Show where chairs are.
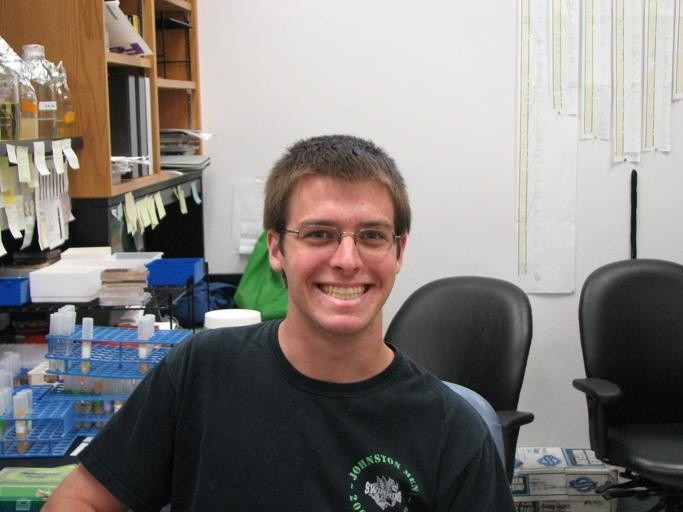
[572,260,683,512]
[384,277,534,486]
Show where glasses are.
[287,225,400,254]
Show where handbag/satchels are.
[235,230,286,320]
[169,282,236,324]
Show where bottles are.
[0,43,58,142]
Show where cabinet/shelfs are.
[0,0,204,199]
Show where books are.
[161,132,211,170]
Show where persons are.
[39,133,518,512]
